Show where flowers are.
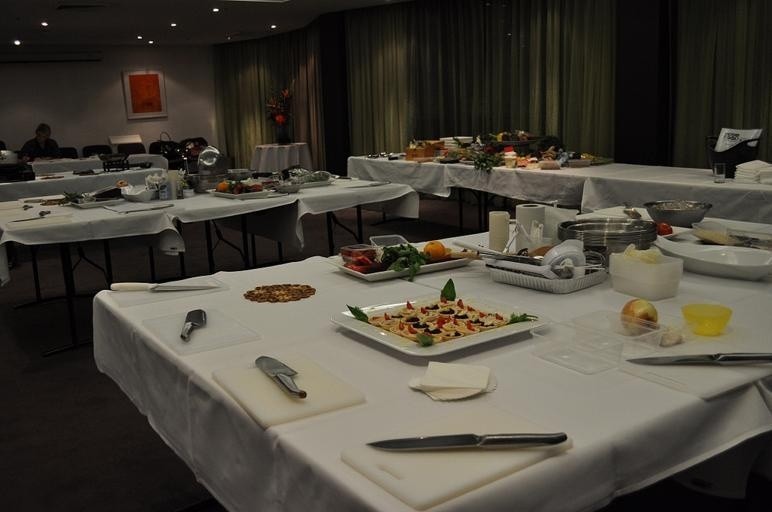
[263,88,294,123]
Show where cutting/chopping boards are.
[108,276,230,307]
[593,205,648,218]
[620,336,772,399]
[5,215,71,230]
[141,307,260,356]
[211,354,365,427]
[0,202,22,211]
[340,402,574,509]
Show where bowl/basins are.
[644,201,713,228]
[680,305,734,339]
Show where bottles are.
[158,168,168,199]
[505,146,517,168]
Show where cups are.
[714,162,727,182]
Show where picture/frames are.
[122,68,168,120]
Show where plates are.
[301,178,335,187]
[440,135,472,154]
[654,235,772,279]
[205,189,274,201]
[121,185,153,202]
[74,195,124,208]
[558,218,657,259]
[329,289,547,358]
[323,254,471,282]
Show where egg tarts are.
[367,297,514,347]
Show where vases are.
[275,123,289,145]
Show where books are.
[714,126,763,152]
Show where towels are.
[734,159,772,185]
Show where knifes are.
[110,282,220,292]
[181,308,206,342]
[255,356,307,402]
[346,180,391,190]
[623,199,641,219]
[624,351,772,367]
[366,432,569,451]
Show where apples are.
[621,298,659,336]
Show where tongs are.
[388,152,404,160]
[460,248,542,265]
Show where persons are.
[18,123,59,163]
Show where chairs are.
[181,137,208,157]
[82,145,113,158]
[117,143,146,155]
[59,146,78,159]
